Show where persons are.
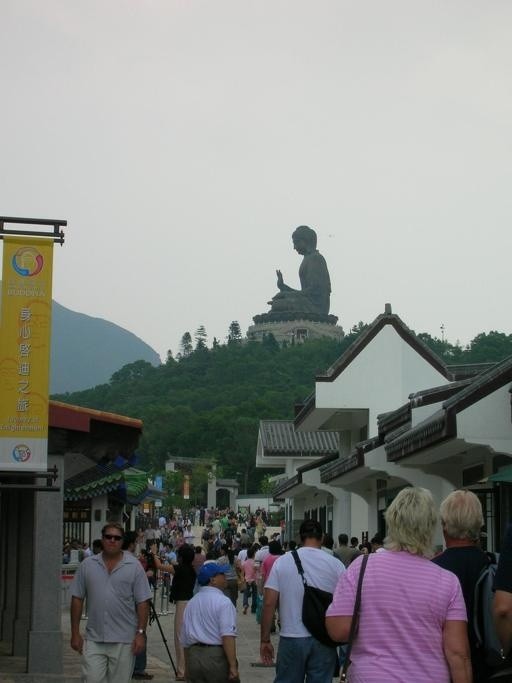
[62,504,364,683]
[269,225,332,313]
[325,487,510,683]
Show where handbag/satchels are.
[237,576,246,592]
[301,585,348,648]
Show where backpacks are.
[471,550,510,668]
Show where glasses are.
[102,534,122,540]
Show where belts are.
[193,642,224,647]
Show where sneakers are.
[242,605,248,614]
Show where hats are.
[197,560,232,584]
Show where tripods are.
[147,582,177,677]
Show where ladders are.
[362,531,368,543]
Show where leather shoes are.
[132,671,154,680]
